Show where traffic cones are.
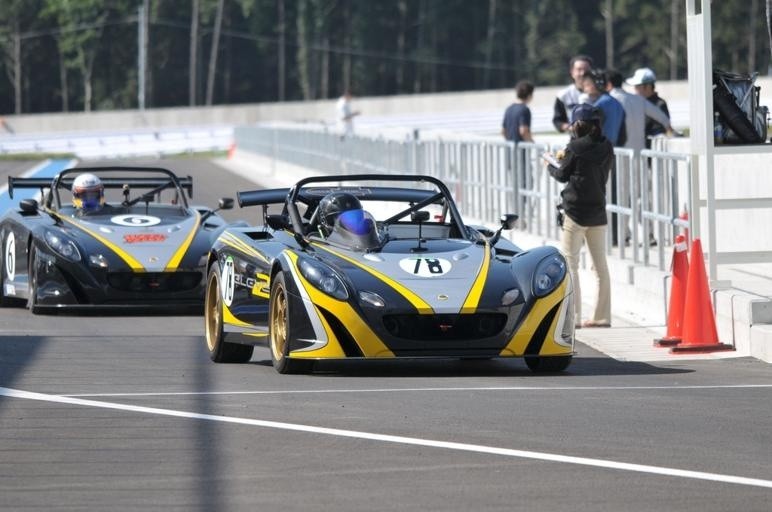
[672,237,735,352]
[654,235,700,346]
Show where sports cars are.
[1,164,248,314]
[200,174,581,376]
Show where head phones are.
[591,68,606,93]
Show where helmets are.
[315,191,362,237]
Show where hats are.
[625,68,656,86]
[567,104,600,127]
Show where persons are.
[316,190,367,244]
[603,71,684,244]
[554,58,595,134]
[538,102,614,328]
[626,68,670,166]
[574,71,626,248]
[502,81,546,208]
